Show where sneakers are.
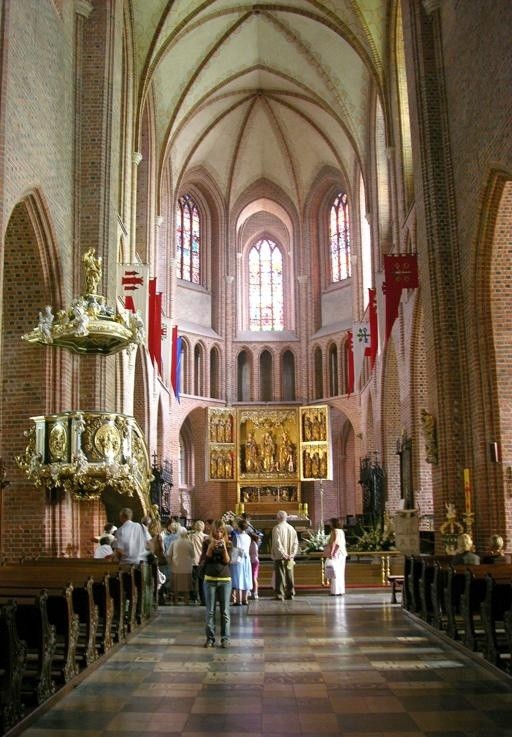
[222,642,230,648]
[204,643,214,648]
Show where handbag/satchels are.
[323,544,340,557]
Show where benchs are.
[388,552,512,666]
[0,557,150,732]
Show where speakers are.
[489,442,499,462]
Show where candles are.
[234,502,240,514]
[303,503,308,514]
[297,501,303,514]
[462,467,472,515]
[320,478,323,484]
[239,503,244,513]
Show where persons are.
[81,246,102,295]
[38,305,55,344]
[198,519,232,649]
[449,533,480,566]
[211,416,328,503]
[323,518,347,596]
[270,510,299,601]
[482,534,504,564]
[93,507,263,606]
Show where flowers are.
[349,521,396,551]
[299,528,328,553]
[220,509,236,522]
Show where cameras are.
[217,541,224,549]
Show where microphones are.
[417,502,420,511]
